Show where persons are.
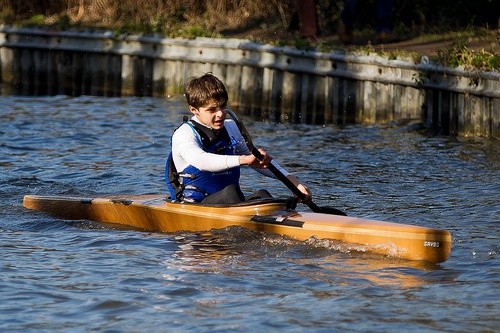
[164,73,313,210]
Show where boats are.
[23,193,450,264]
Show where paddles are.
[226,109,348,216]
